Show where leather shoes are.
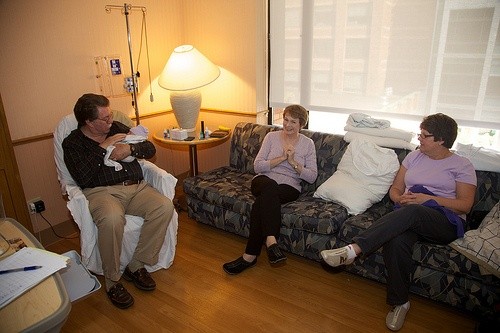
[105,282,134,309]
[122,262,156,291]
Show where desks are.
[0,217,103,333]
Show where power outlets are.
[26,197,42,215]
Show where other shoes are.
[386,300,411,331]
[222,254,257,275]
[321,246,356,268]
[266,243,289,264]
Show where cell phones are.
[184,137,195,141]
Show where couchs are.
[183,118,500,325]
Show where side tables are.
[152,129,232,211]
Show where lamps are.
[159,44,221,131]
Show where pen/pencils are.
[0,265,43,275]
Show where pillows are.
[313,140,401,216]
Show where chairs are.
[53,110,180,278]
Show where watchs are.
[129,143,135,154]
[293,164,299,169]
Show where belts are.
[113,178,143,186]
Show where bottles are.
[163,126,188,140]
[199,126,211,140]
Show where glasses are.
[96,111,113,122]
[417,133,435,139]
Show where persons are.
[222,106,319,275]
[320,113,477,331]
[61,94,175,308]
